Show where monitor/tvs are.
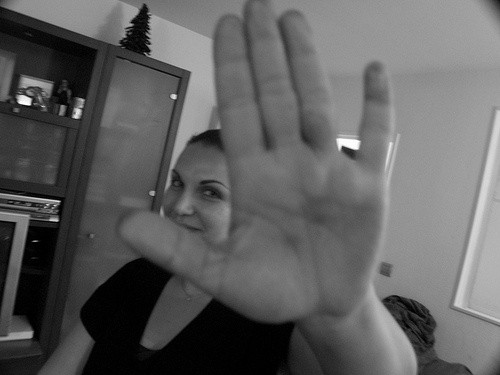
[0,211,29,337]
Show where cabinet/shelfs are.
[0,7,191,375]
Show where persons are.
[385,293,475,375]
[38,0,419,375]
